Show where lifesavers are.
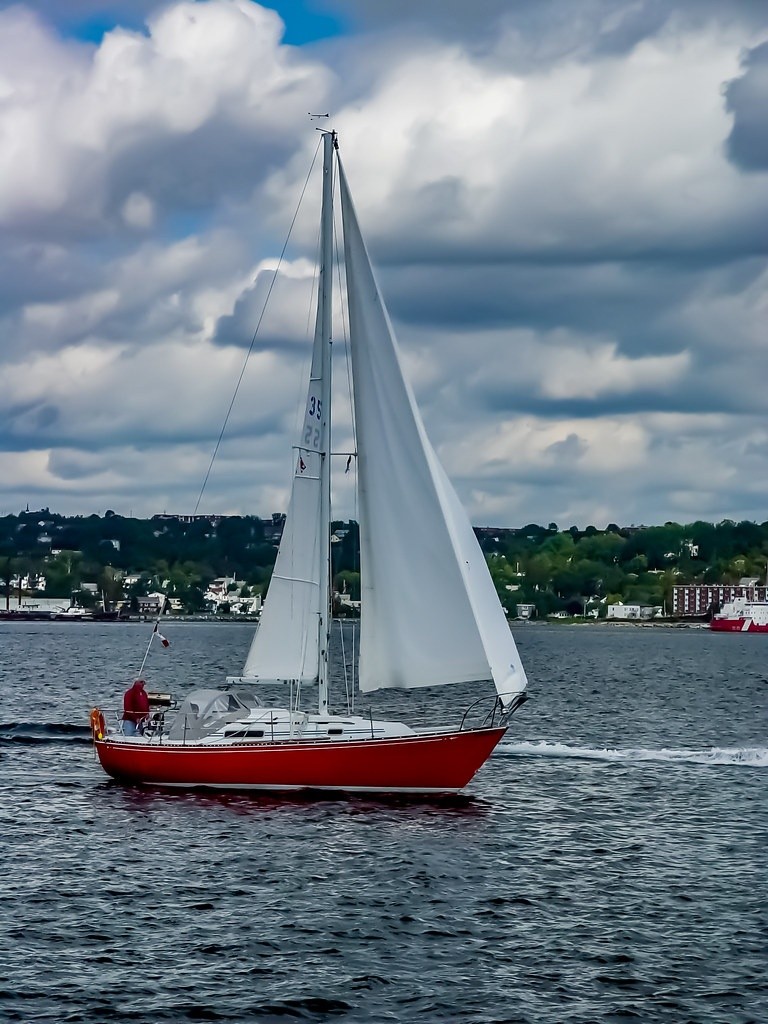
[90,708,105,739]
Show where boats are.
[0,602,122,622]
[710,594,768,632]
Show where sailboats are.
[89,112,531,795]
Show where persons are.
[119,678,151,738]
